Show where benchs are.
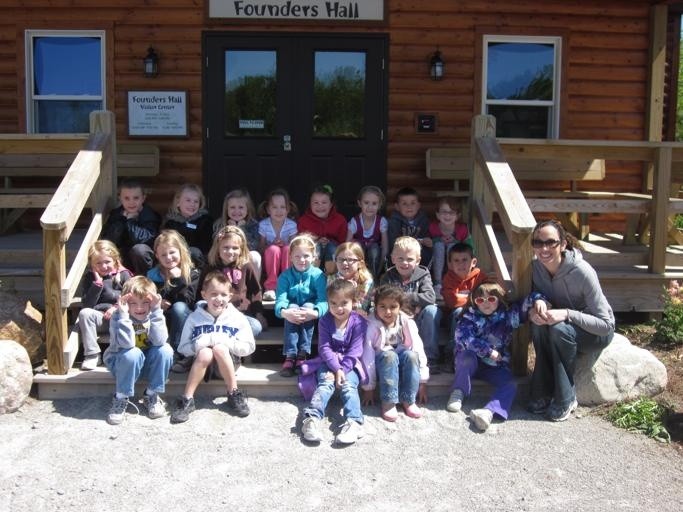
[0,133,159,240]
[427,146,606,237]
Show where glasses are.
[475,296,498,304]
[530,238,558,249]
[336,256,359,263]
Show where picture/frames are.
[126,88,190,138]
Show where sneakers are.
[335,417,363,444]
[227,388,250,417]
[446,388,465,411]
[428,356,455,374]
[170,394,195,423]
[526,394,552,414]
[470,407,493,431]
[434,283,444,300]
[171,355,193,373]
[280,350,309,378]
[143,388,166,418]
[107,391,129,424]
[381,401,398,422]
[400,398,422,418]
[263,289,276,301]
[81,353,103,369]
[545,395,579,422]
[300,416,323,441]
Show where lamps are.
[429,44,444,80]
[143,47,158,77]
